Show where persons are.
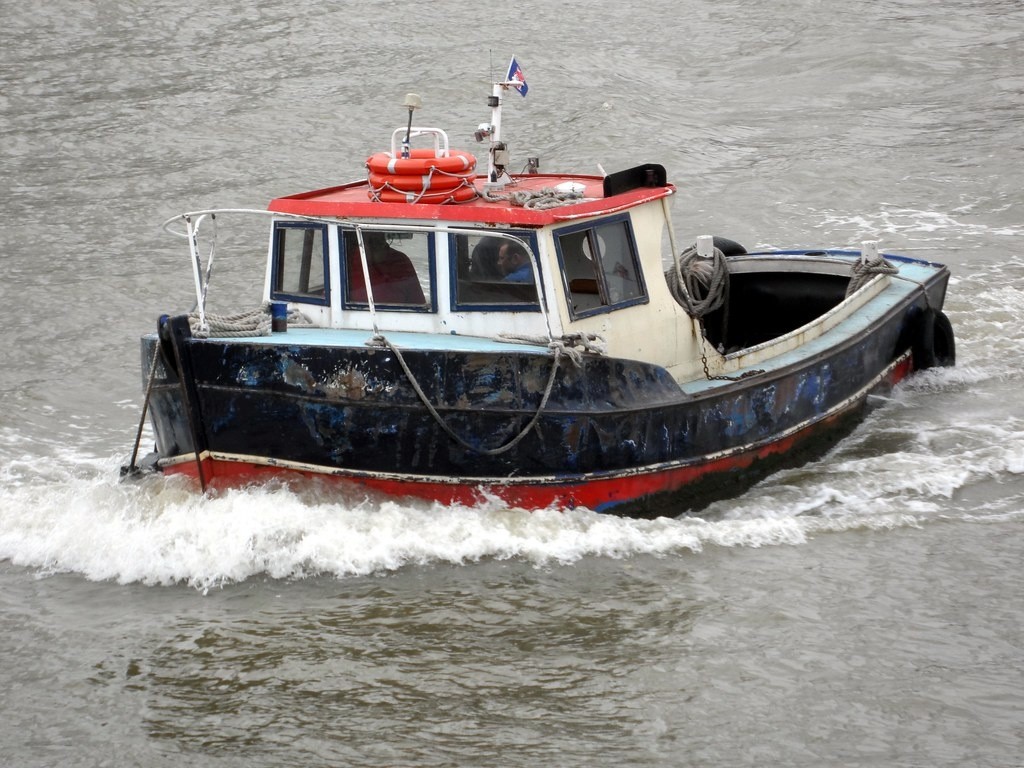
[347,231,428,313]
[498,242,535,283]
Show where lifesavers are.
[365,170,476,190]
[680,235,747,256]
[368,187,477,205]
[365,148,477,175]
[912,308,955,370]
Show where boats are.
[118,49,956,525]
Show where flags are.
[509,59,529,97]
